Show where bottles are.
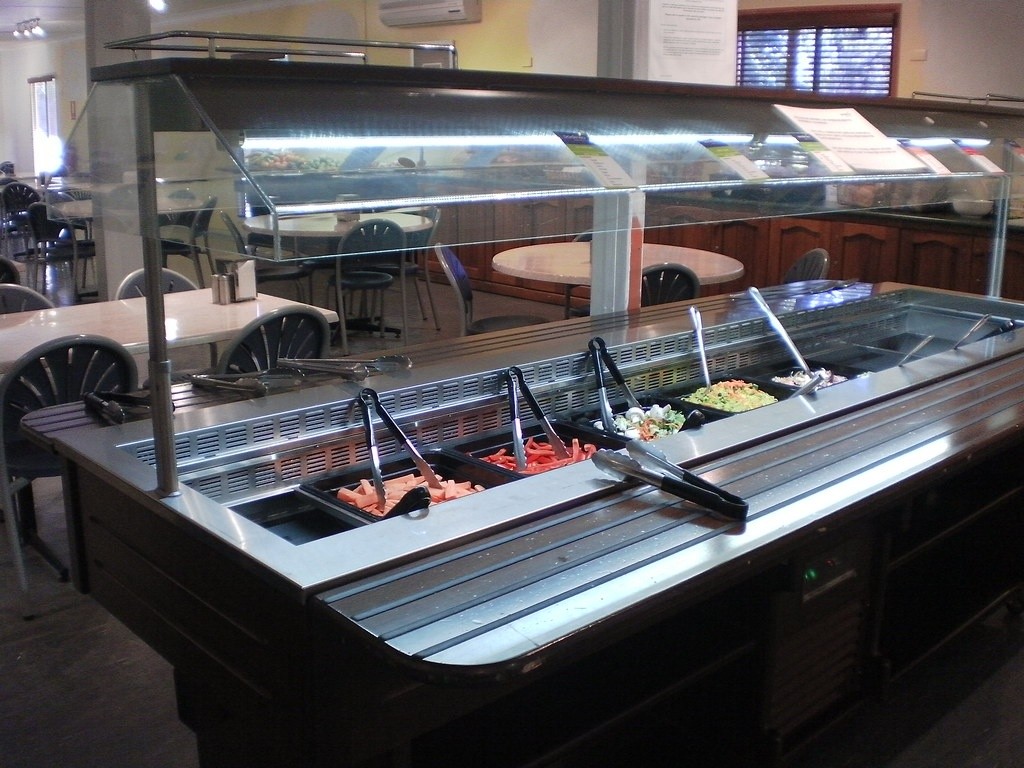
[211,274,234,305]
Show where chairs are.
[140,305,331,389]
[0,332,138,481]
[219,210,315,305]
[782,246,831,285]
[359,205,441,332]
[562,229,594,319]
[433,243,550,337]
[326,218,408,355]
[641,262,702,307]
[0,171,218,315]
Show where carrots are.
[483,435,596,473]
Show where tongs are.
[277,355,412,382]
[976,320,1016,341]
[84,391,176,425]
[382,486,432,520]
[358,388,443,514]
[588,337,642,433]
[678,410,705,433]
[505,367,570,469]
[592,439,749,519]
[190,368,307,398]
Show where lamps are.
[12,17,40,37]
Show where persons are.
[0,161,19,191]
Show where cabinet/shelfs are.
[415,181,1024,312]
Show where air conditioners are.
[378,0,482,28]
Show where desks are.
[0,176,85,260]
[491,240,744,318]
[51,197,203,297]
[240,214,433,339]
[0,288,340,584]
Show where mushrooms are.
[625,407,645,422]
[650,404,671,420]
